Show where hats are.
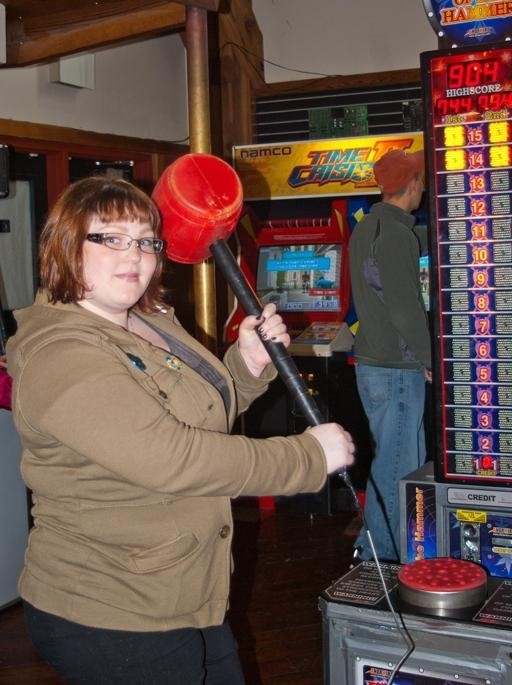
[372,148,425,193]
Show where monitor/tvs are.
[419,255,430,312]
[256,243,343,313]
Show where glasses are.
[81,230,165,256]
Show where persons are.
[301,271,311,294]
[3,173,359,685]
[314,276,334,300]
[418,267,429,292]
[347,146,433,574]
